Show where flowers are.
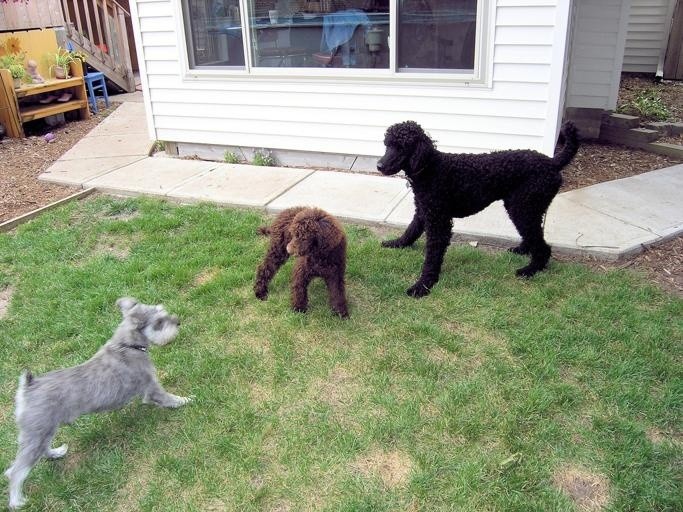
[0,35,33,84]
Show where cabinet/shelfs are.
[0,26,91,138]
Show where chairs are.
[256,26,312,66]
[309,22,364,68]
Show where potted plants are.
[45,46,87,79]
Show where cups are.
[268,10,279,25]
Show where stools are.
[83,72,110,114]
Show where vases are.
[12,77,20,88]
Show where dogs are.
[252,204,350,321]
[374,119,583,300]
[3,295,198,512]
